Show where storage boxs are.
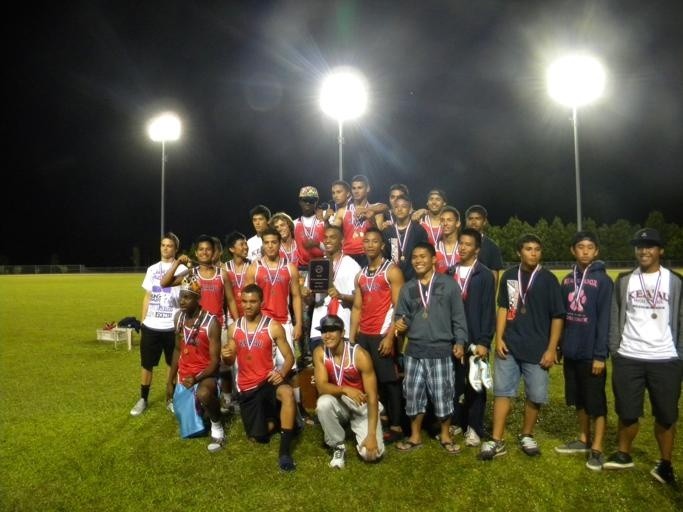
[97,327,128,341]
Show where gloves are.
[555,346,563,364]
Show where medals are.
[651,313,657,319]
[520,307,527,314]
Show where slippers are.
[208,430,225,451]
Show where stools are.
[113,325,141,352]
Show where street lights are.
[314,60,372,184]
[140,106,183,242]
[545,50,611,227]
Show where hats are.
[631,228,662,246]
[573,231,598,246]
[300,186,318,198]
[180,277,202,296]
[314,314,344,330]
[428,188,447,200]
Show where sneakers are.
[279,455,296,472]
[384,425,480,454]
[296,401,315,425]
[220,393,240,414]
[555,439,634,470]
[130,398,147,416]
[518,431,541,456]
[167,403,175,414]
[469,355,493,392]
[651,458,678,491]
[478,439,507,459]
[328,442,345,469]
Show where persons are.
[552,232,615,472]
[476,234,567,460]
[129,175,503,471]
[600,226,682,494]
